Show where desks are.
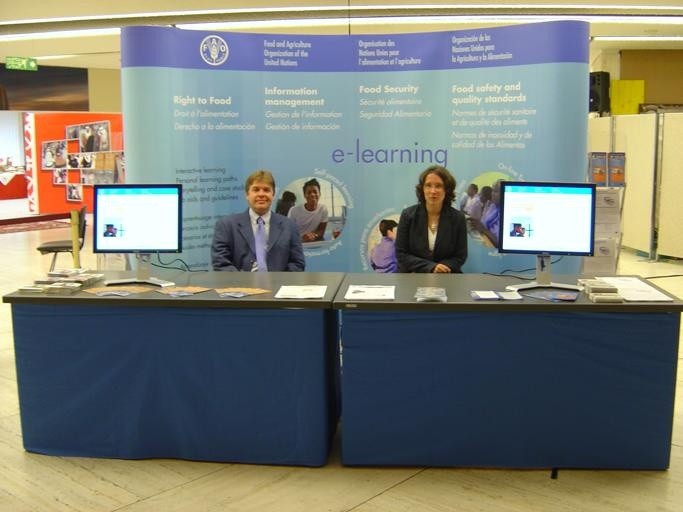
[3,270,346,467]
[333,272,683,470]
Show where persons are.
[287,179,329,244]
[209,169,306,273]
[508,222,528,237]
[275,191,296,216]
[462,178,503,248]
[103,224,117,237]
[393,163,469,273]
[41,121,125,205]
[367,218,399,273]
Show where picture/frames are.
[41,120,124,201]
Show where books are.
[579,284,622,304]
[576,276,619,294]
[16,268,176,302]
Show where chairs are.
[36,206,87,272]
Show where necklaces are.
[425,206,440,232]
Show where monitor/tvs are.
[93,184,182,288]
[498,181,596,291]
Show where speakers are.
[589,71,610,112]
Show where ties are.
[255,218,267,272]
